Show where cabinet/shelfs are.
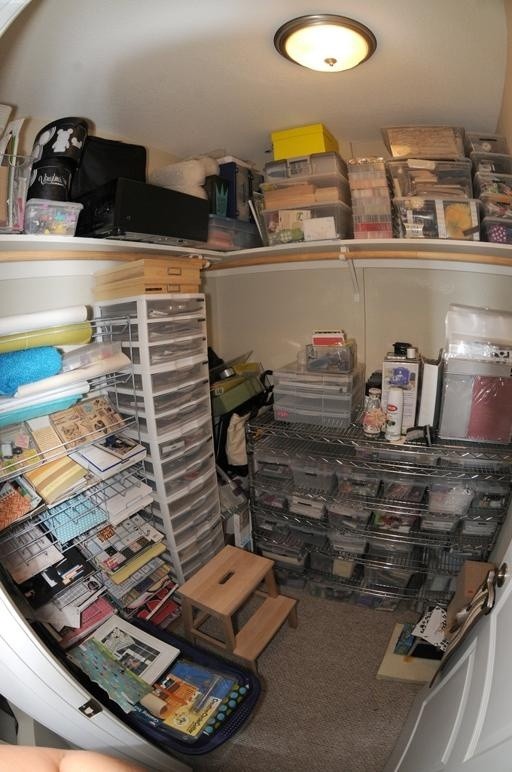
[247,301,512,609]
[0,295,260,772]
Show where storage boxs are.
[0,103,512,302]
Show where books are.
[27,437,149,502]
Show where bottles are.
[384,386,404,441]
[363,387,383,438]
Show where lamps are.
[275,15,377,73]
